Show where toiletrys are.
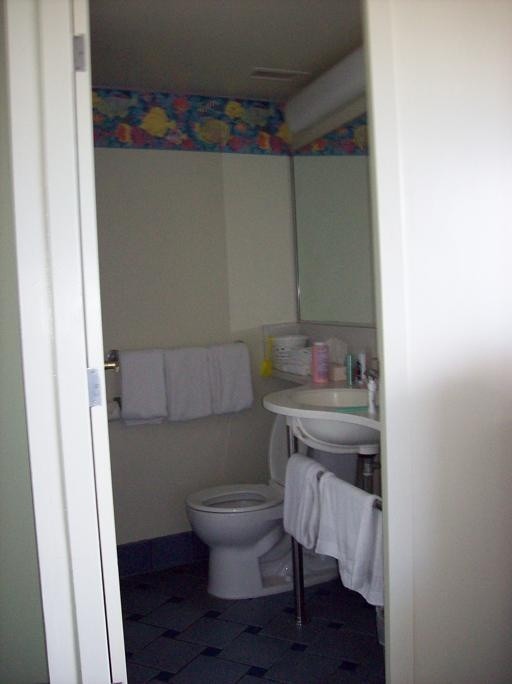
[367,375,377,415]
[346,351,368,384]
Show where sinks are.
[263,387,379,456]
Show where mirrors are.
[291,111,374,329]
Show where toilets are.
[185,414,358,600]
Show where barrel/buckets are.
[270,334,310,369]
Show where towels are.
[118,337,253,428]
[281,452,385,606]
